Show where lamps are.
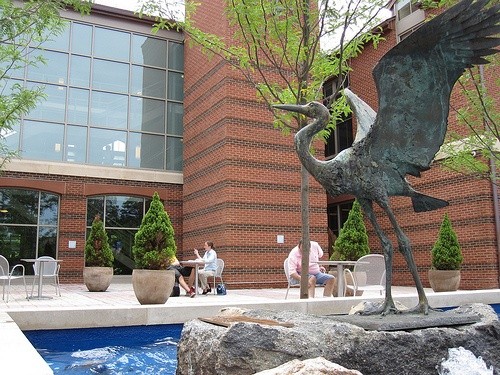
[0,193,10,212]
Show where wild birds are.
[270,0,500,318]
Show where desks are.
[18,258,63,300]
[180,260,214,297]
[310,260,370,297]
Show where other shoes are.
[202,285,212,295]
[186,287,196,296]
[187,288,196,298]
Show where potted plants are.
[328,204,368,296]
[83,213,113,291]
[429,213,463,293]
[132,191,176,304]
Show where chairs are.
[284,258,328,299]
[31,256,61,297]
[0,255,29,303]
[344,254,388,297]
[212,259,226,295]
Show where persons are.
[288,239,335,297]
[170,256,196,298]
[193,241,217,294]
